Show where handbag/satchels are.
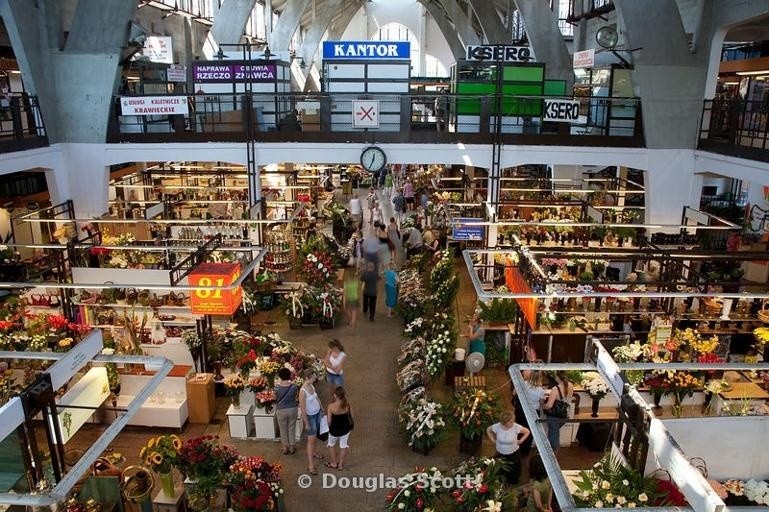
[347,255,356,267]
[324,351,332,383]
[320,414,330,435]
[397,228,401,240]
[317,427,328,441]
[382,188,387,196]
[348,403,354,429]
[542,385,569,420]
[75,282,187,307]
[402,196,407,213]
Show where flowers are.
[8,329,30,344]
[645,373,674,397]
[223,375,249,395]
[397,386,450,449]
[583,379,610,401]
[101,363,120,392]
[443,387,504,441]
[140,434,182,473]
[255,388,278,412]
[257,268,274,295]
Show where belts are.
[306,408,322,417]
[325,369,340,377]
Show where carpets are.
[28,391,222,500]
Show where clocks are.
[359,146,386,172]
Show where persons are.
[360,262,379,322]
[392,190,406,230]
[323,385,355,471]
[353,231,364,276]
[373,220,380,234]
[543,371,574,456]
[273,368,300,456]
[323,338,348,387]
[403,179,415,211]
[366,229,382,280]
[424,230,441,257]
[387,217,401,264]
[298,369,325,476]
[433,90,449,132]
[383,263,401,319]
[406,223,423,257]
[516,370,546,457]
[378,224,388,244]
[342,267,361,330]
[333,185,343,203]
[307,222,317,240]
[464,316,486,355]
[366,186,378,223]
[383,170,394,201]
[421,190,429,205]
[349,194,363,231]
[486,411,530,485]
[369,201,384,227]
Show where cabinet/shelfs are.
[186,374,217,424]
[536,299,667,334]
[710,382,769,415]
[545,323,632,362]
[670,296,762,335]
[225,403,252,440]
[574,383,587,393]
[721,495,768,512]
[238,387,256,426]
[253,406,277,442]
[0,265,206,374]
[507,322,551,364]
[261,220,295,281]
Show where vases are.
[591,401,599,417]
[160,471,175,497]
[412,446,430,457]
[265,404,272,415]
[260,294,274,311]
[108,382,122,420]
[11,340,28,352]
[151,464,173,479]
[654,393,663,415]
[458,429,483,455]
[232,395,240,409]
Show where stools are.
[153,487,185,511]
[295,408,305,441]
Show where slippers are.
[290,446,297,455]
[337,463,344,472]
[313,452,324,460]
[324,461,338,470]
[282,447,289,455]
[307,465,316,476]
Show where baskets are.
[92,457,122,477]
[120,464,154,505]
[757,301,769,324]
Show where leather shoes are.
[369,316,375,323]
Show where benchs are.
[10,370,188,433]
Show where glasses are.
[473,314,479,317]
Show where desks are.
[564,407,622,444]
[138,337,197,374]
